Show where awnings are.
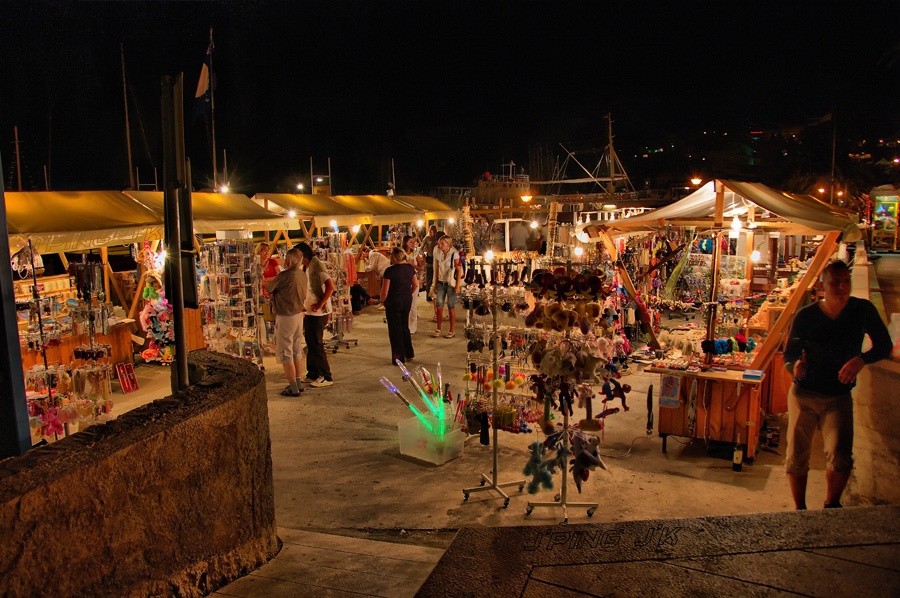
[3,191,456,255]
[579,177,861,243]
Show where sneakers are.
[311,376,334,387]
[301,376,313,382]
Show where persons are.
[266,248,308,397]
[351,284,378,315]
[420,225,461,339]
[294,242,334,388]
[255,243,283,351]
[401,235,423,334]
[379,248,417,364]
[362,247,390,310]
[783,261,893,509]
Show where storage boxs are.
[397,414,465,465]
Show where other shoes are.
[427,298,432,302]
[281,385,304,396]
[433,316,438,322]
[445,331,454,337]
[433,330,441,336]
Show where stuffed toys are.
[134,242,175,364]
[27,396,96,439]
[521,267,633,495]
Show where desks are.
[644,363,766,464]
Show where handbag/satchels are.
[448,252,465,288]
